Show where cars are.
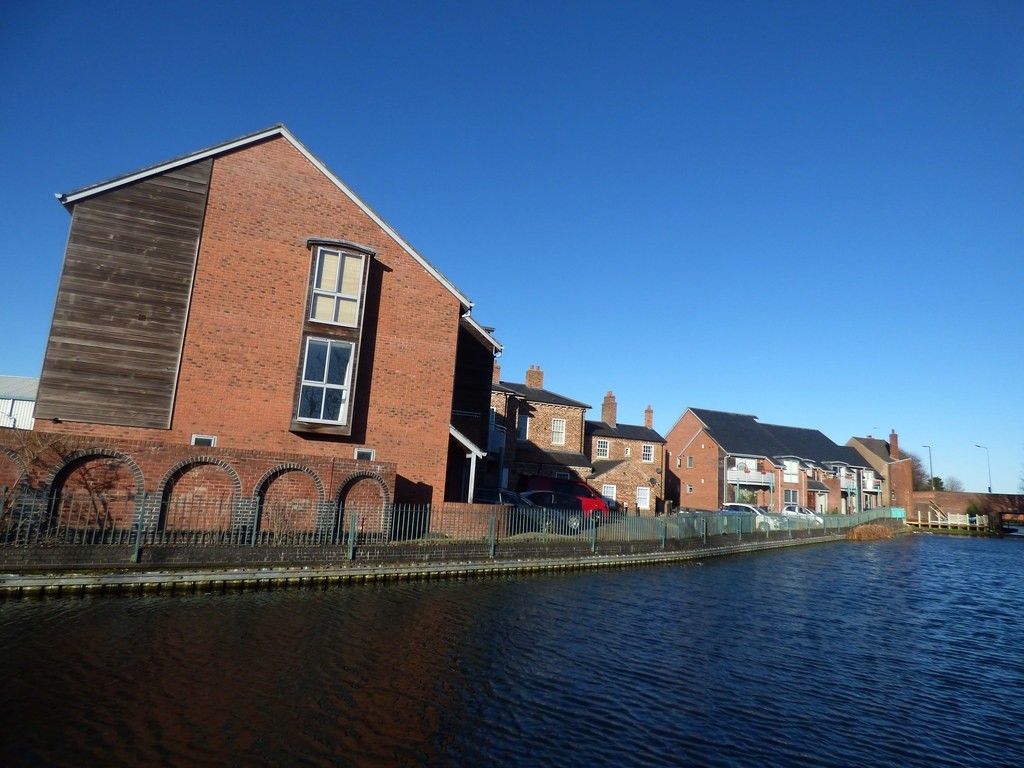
[473,487,559,535]
[671,506,713,518]
[516,491,583,538]
[513,478,611,525]
[781,503,825,528]
[719,502,784,533]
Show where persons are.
[623,499,628,516]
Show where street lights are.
[923,444,935,490]
[974,441,991,492]
